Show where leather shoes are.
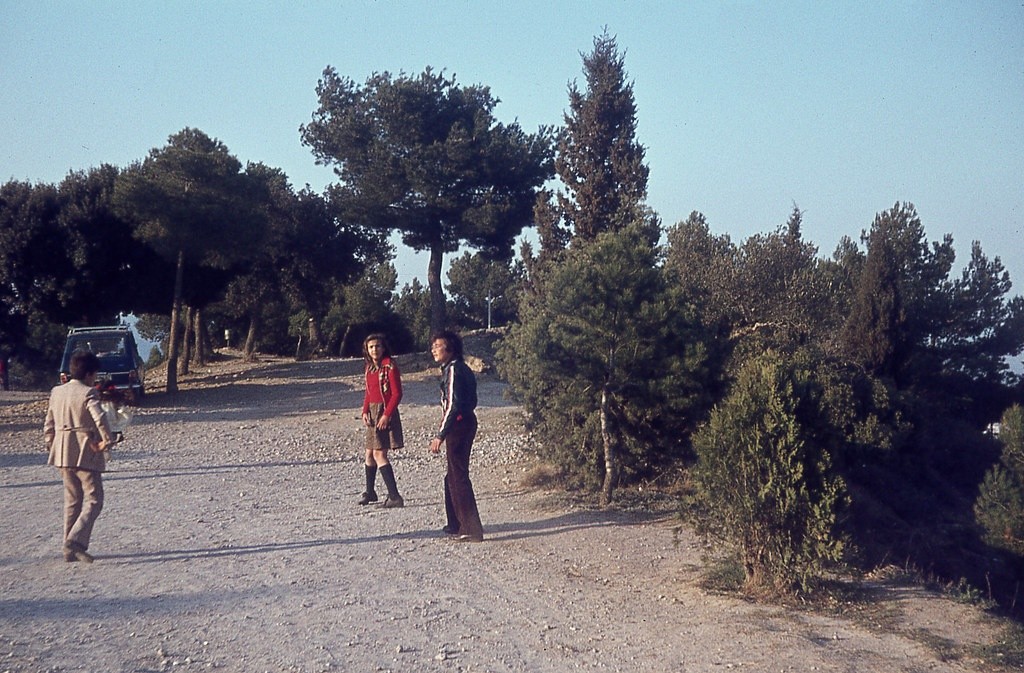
[358,489,403,508]
[443,525,483,542]
[62,539,95,562]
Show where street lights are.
[485,289,496,330]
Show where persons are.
[430,330,483,543]
[358,333,407,508]
[43,351,114,562]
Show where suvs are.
[60,326,148,405]
